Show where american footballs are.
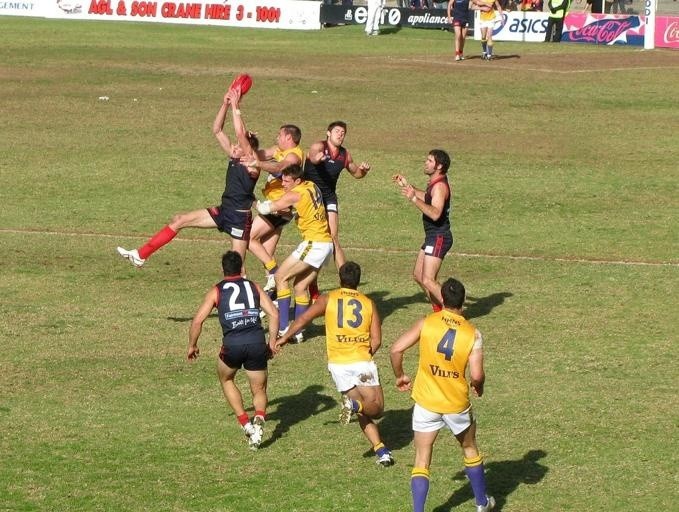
[230,71,253,95]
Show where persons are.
[468,1,504,59]
[445,1,474,61]
[543,0,572,42]
[389,277,497,512]
[251,164,335,344]
[303,121,371,305]
[399,0,641,15]
[117,77,261,281]
[240,125,304,307]
[365,0,386,36]
[392,141,454,316]
[273,261,395,468]
[185,251,280,449]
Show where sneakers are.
[475,494,496,511]
[455,54,460,60]
[460,54,465,60]
[338,394,353,425]
[481,52,492,62]
[288,328,306,344]
[241,420,254,436]
[263,274,278,292]
[249,415,265,451]
[375,446,394,467]
[268,324,289,340]
[372,30,381,36]
[117,247,146,268]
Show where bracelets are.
[410,196,419,204]
[234,109,241,115]
